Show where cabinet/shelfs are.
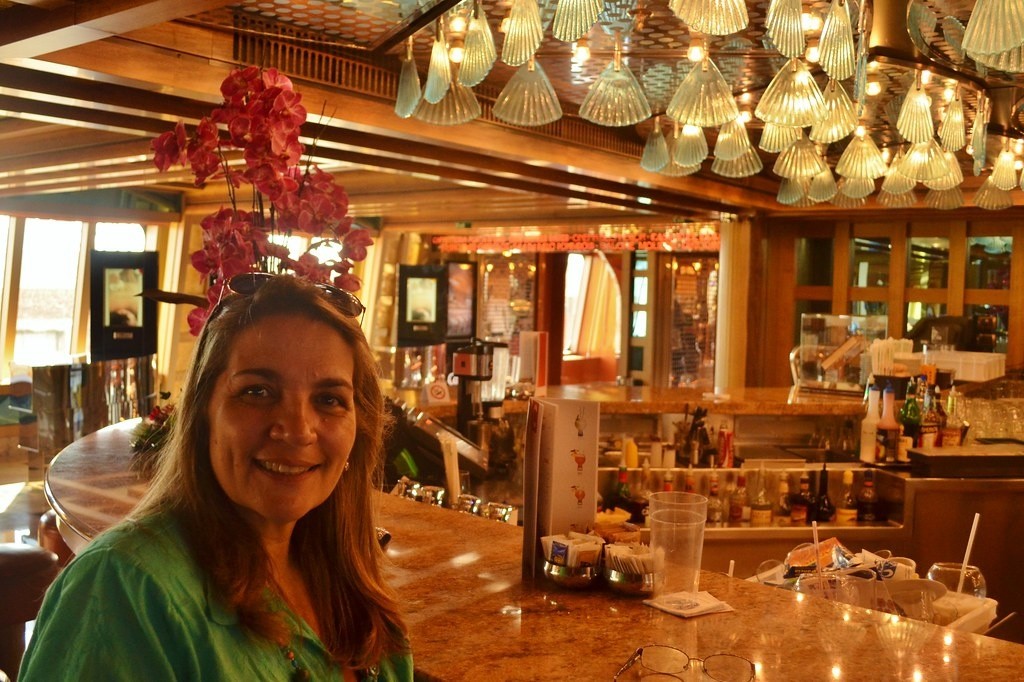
[534,385,902,529]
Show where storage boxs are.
[908,443,1024,480]
[744,563,998,636]
[894,350,1007,382]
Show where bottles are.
[857,482,878,521]
[626,438,638,468]
[836,469,858,525]
[650,418,733,468]
[814,470,834,521]
[706,472,751,522]
[860,376,969,462]
[616,463,695,502]
[751,468,814,525]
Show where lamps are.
[393,1,1024,213]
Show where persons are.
[16,272,413,682]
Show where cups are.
[756,543,987,624]
[923,345,956,389]
[961,398,1024,431]
[649,492,708,609]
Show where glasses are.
[201,273,366,333]
[613,645,755,682]
[756,543,815,582]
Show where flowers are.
[151,64,376,336]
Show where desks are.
[379,377,457,429]
[637,511,902,579]
